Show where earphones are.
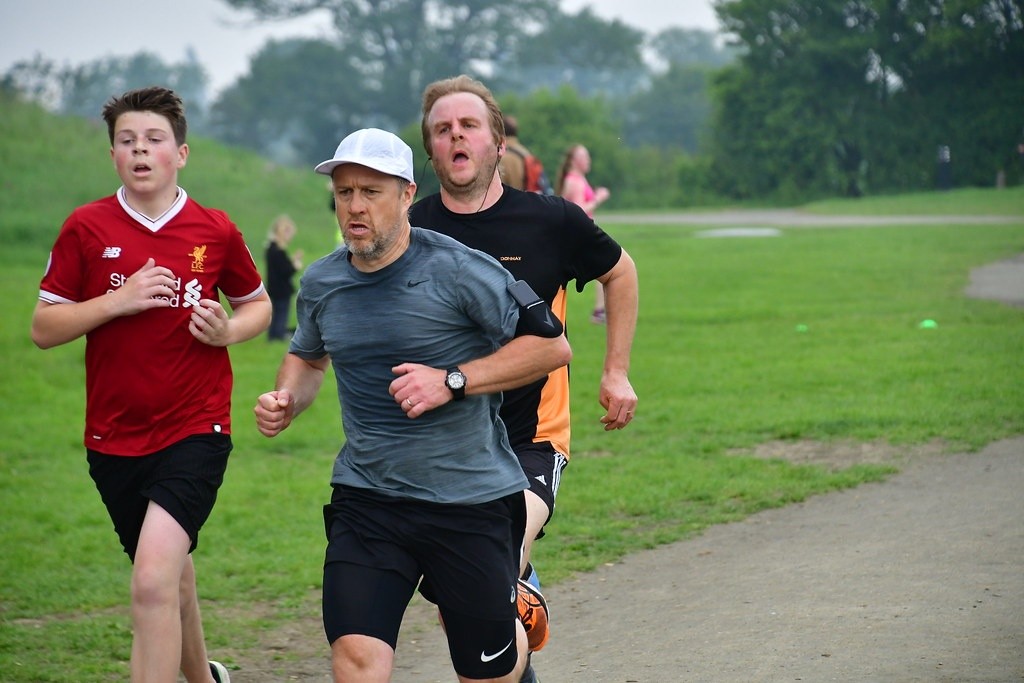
[501,145,502,154]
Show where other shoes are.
[208,660,231,683]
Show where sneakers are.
[515,579,550,652]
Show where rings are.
[406,399,413,407]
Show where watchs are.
[444,365,467,402]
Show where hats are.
[314,128,415,184]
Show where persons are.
[32,87,273,683]
[261,215,303,341]
[252,128,572,683]
[498,116,611,322]
[402,75,639,651]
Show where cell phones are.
[507,280,540,307]
